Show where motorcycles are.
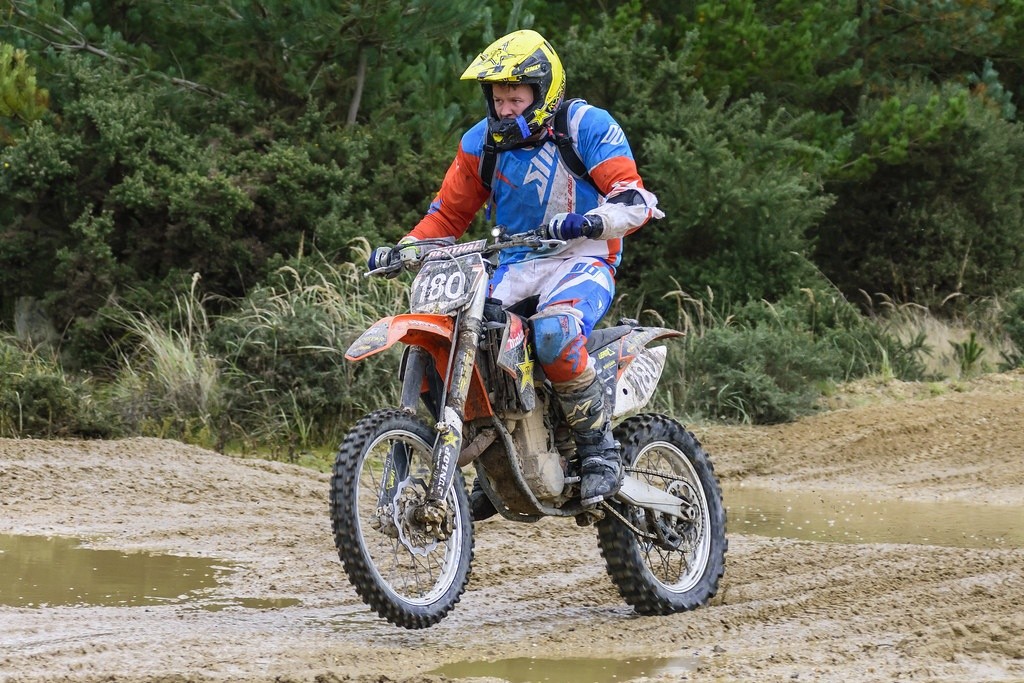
[327,218,729,628]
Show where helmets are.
[460,30,567,151]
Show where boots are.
[557,374,625,507]
[469,477,498,521]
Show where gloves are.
[368,247,402,279]
[547,212,591,247]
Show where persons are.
[359,26,656,524]
